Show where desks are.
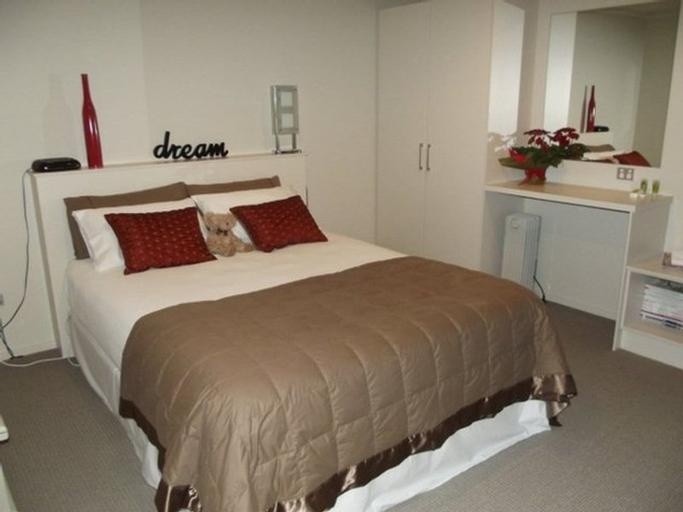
[484,179,673,351]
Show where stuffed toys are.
[203,211,254,257]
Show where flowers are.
[497,127,562,168]
[547,128,592,160]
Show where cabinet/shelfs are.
[376,0,525,273]
[618,259,683,371]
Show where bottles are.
[586,84,597,131]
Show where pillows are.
[63,174,328,276]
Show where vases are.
[587,85,596,132]
[518,168,546,186]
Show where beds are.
[25,150,577,512]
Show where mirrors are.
[542,0,681,168]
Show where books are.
[639,282,683,331]
